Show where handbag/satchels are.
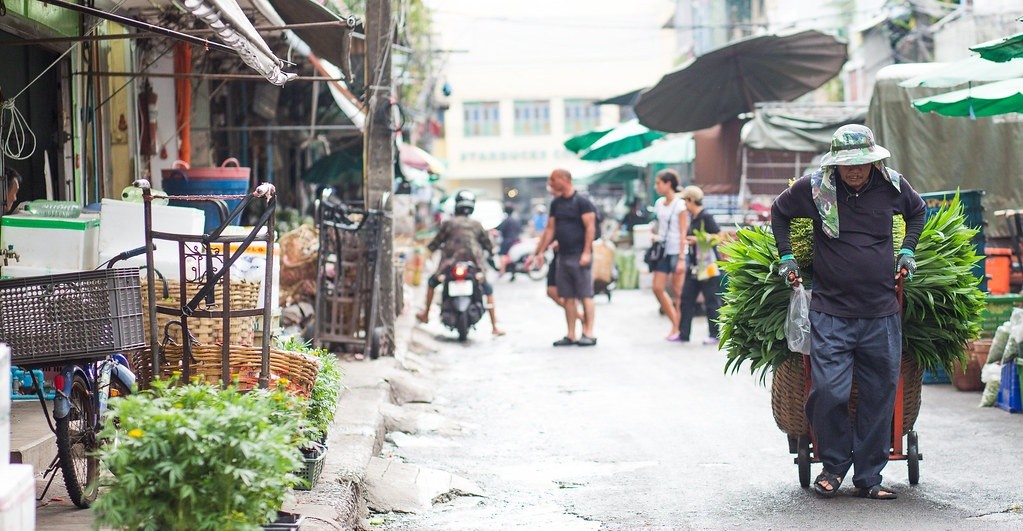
[784,283,812,355]
[695,235,720,281]
[644,241,666,272]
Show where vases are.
[954,338,993,390]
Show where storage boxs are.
[632,223,653,247]
[995,357,1023,413]
[0,209,280,380]
[922,360,948,384]
[918,188,1023,338]
[0,462,35,531]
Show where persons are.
[523,168,597,346]
[650,167,691,340]
[668,186,725,345]
[3,164,21,213]
[486,200,521,282]
[415,188,505,335]
[526,191,650,235]
[771,124,927,499]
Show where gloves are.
[778,256,802,285]
[896,249,917,282]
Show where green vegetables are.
[711,186,991,381]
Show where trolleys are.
[305,200,384,361]
[786,268,923,487]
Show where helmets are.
[455,189,476,214]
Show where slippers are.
[859,484,897,499]
[813,468,845,495]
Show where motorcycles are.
[434,246,492,340]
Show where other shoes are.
[665,330,680,341]
[671,336,687,343]
[552,337,577,346]
[575,335,597,345]
[703,337,720,345]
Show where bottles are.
[24,200,81,218]
[121,186,169,206]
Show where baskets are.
[276,224,322,306]
[130,321,317,406]
[0,267,146,367]
[161,157,250,234]
[130,282,260,344]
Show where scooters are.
[485,231,553,281]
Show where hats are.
[810,124,901,240]
[677,186,703,200]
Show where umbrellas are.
[563,30,849,196]
[898,14,1023,119]
[298,140,443,188]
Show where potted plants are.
[80,337,347,531]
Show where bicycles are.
[0,242,157,509]
